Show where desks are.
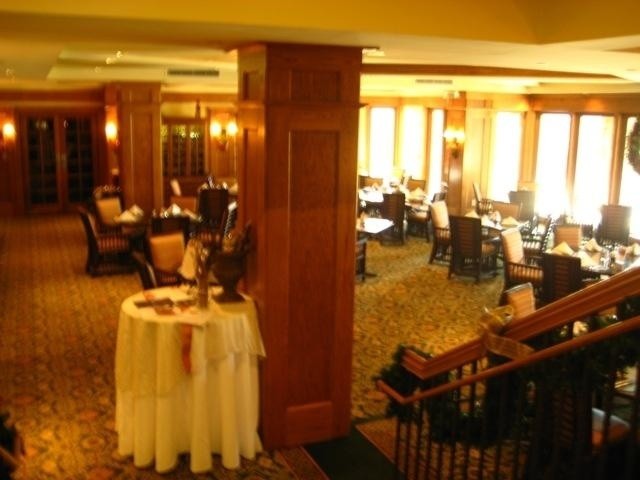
[119,289,261,475]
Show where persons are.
[0,405,27,480]
